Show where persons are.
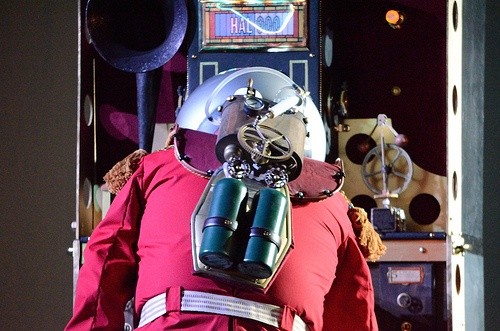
[63,65,380,331]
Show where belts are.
[136,291,311,331]
